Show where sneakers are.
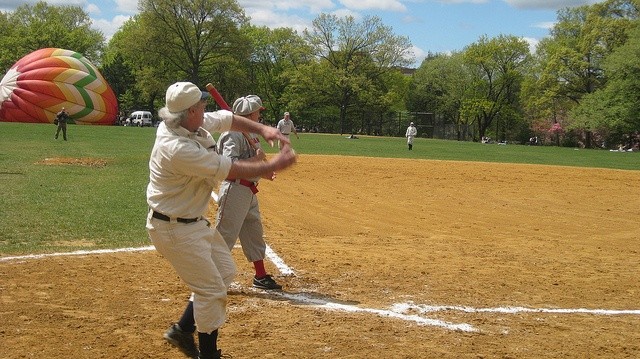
[252,275,282,289]
[162,323,197,358]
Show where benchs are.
[529,136,537,144]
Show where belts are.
[282,133,289,135]
[147,206,203,223]
[227,178,259,195]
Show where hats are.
[284,112,290,115]
[410,122,414,125]
[232,94,267,116]
[165,81,209,113]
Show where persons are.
[214,94,283,292]
[276,112,300,152]
[146,81,298,359]
[481,135,508,146]
[529,136,539,146]
[610,142,640,152]
[258,118,277,128]
[355,128,384,135]
[405,121,417,151]
[53,107,69,141]
[294,124,328,132]
[117,110,136,126]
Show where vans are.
[126,111,152,127]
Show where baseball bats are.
[206,83,267,162]
